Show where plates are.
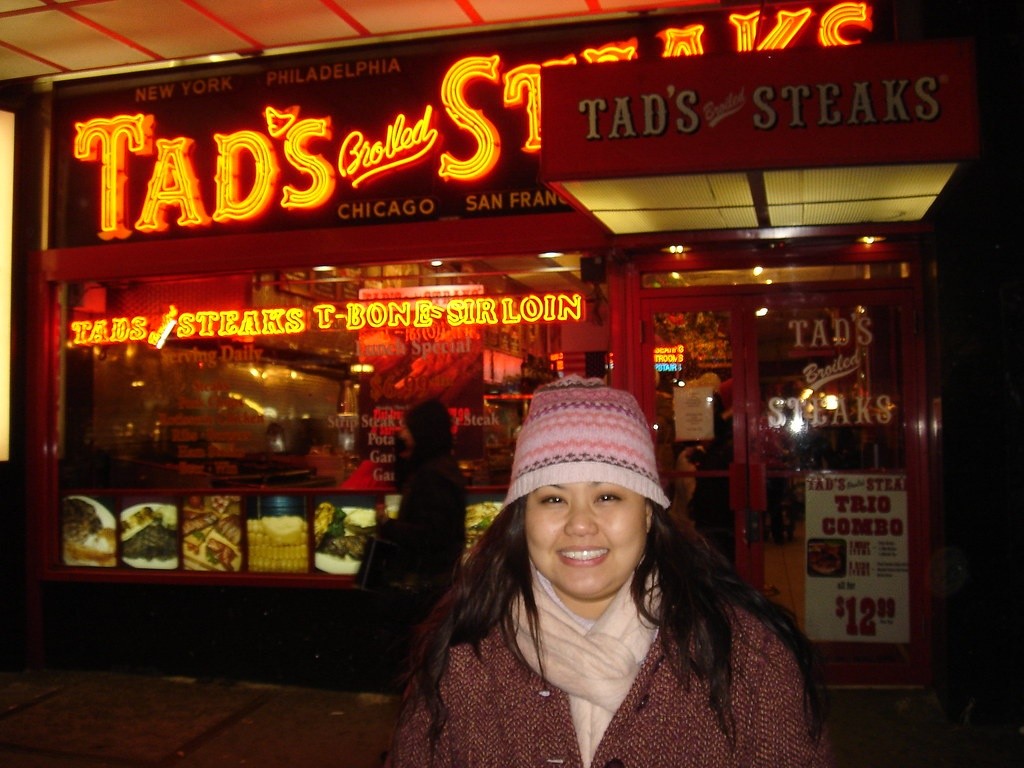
[807,541,842,573]
[311,499,382,574]
[55,494,243,575]
[461,499,506,566]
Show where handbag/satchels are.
[353,537,399,590]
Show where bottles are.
[336,380,359,449]
[502,354,559,395]
[333,440,360,485]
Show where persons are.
[389,372,835,768]
[655,388,734,565]
[762,412,797,544]
[372,400,468,760]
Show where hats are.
[501,374,670,510]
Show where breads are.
[66,527,117,563]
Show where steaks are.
[317,533,369,560]
[117,524,181,561]
